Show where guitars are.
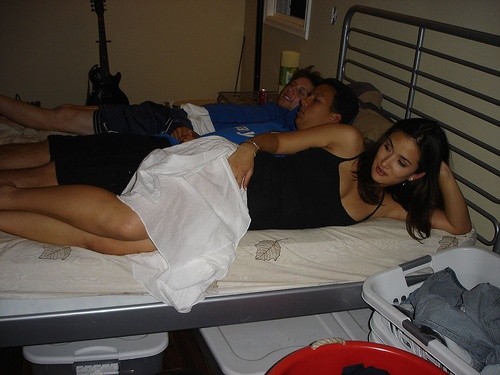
[84,0,130,105]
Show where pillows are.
[345,80,396,145]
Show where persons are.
[0,66,360,196]
[0,118,472,256]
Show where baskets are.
[361,243,500,375]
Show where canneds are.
[259,89,269,104]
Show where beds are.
[1,4,499,354]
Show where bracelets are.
[236,139,260,157]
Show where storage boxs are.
[22,331,172,375]
[361,246,499,375]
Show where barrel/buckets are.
[266,341,451,375]
